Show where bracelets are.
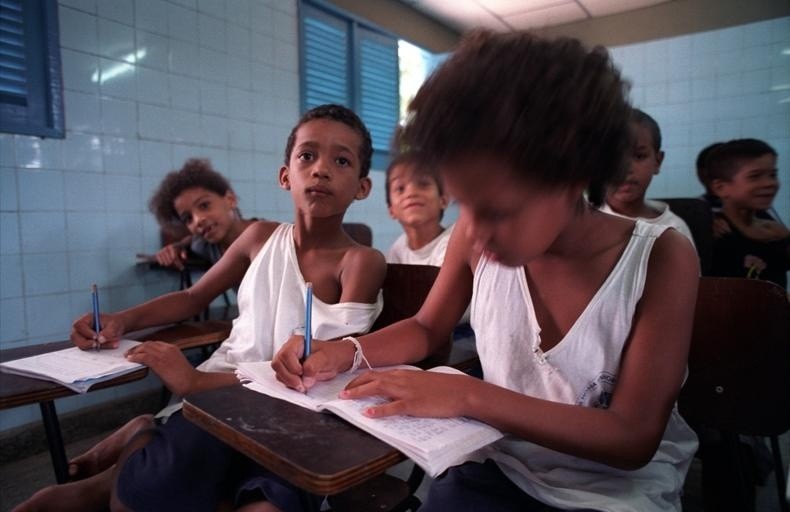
[342,336,373,374]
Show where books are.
[234,358,509,479]
[0,339,148,394]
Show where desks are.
[181,383,424,510]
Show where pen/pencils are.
[304,281,313,395]
[92,283,101,350]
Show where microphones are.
[151,237,210,273]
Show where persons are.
[271,25,700,511]
[0,104,388,512]
[694,138,790,291]
[385,155,474,326]
[146,158,268,295]
[695,143,775,221]
[583,109,697,252]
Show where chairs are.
[653,198,721,271]
[676,275,790,512]
[367,264,454,371]
[337,224,375,247]
